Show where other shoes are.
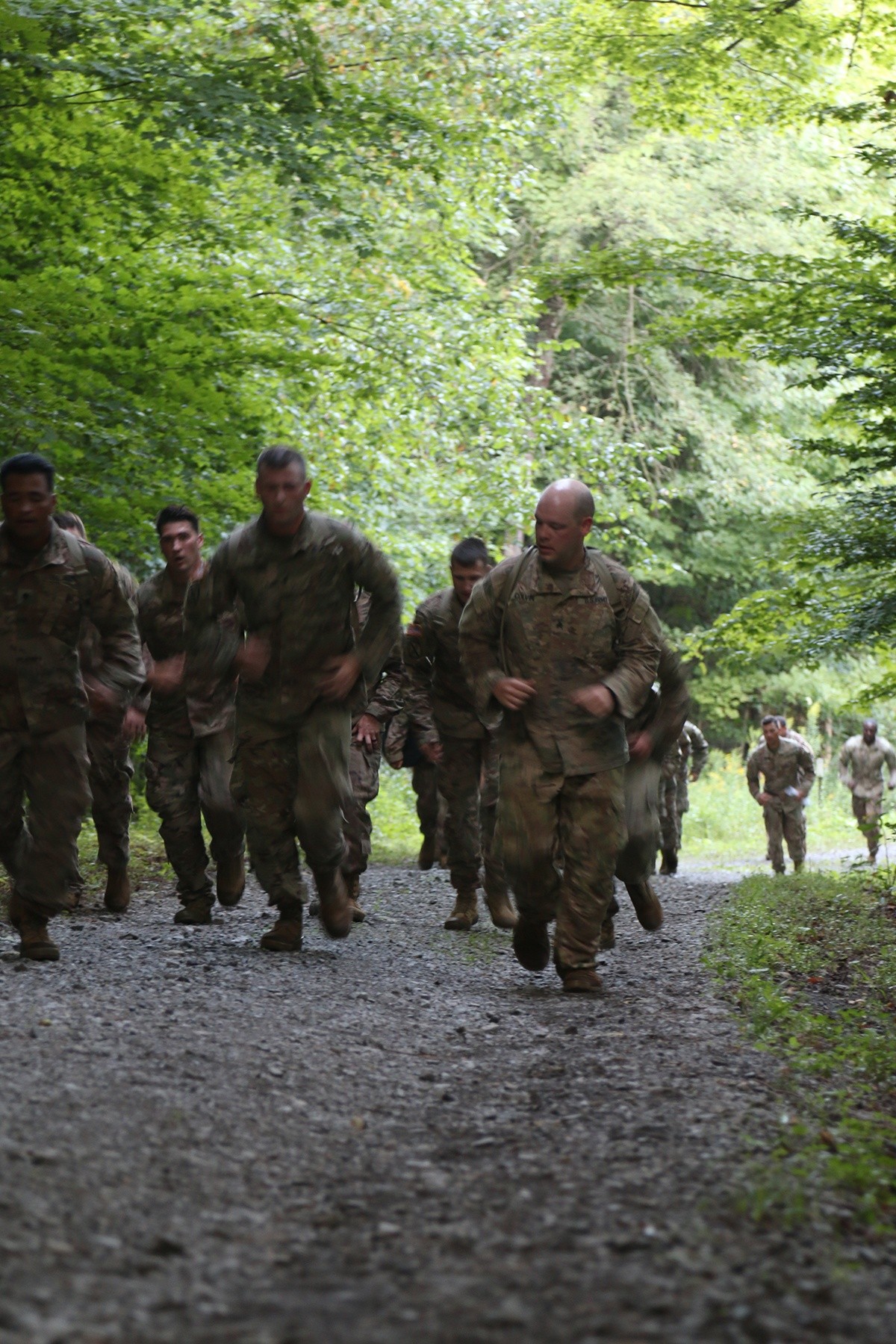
[625,881,663,931]
[7,909,60,962]
[444,891,479,929]
[659,853,679,874]
[599,919,616,950]
[485,890,518,928]
[260,920,304,951]
[313,867,353,939]
[608,897,620,918]
[562,968,604,993]
[174,899,212,925]
[216,862,246,906]
[105,869,130,909]
[350,900,365,923]
[514,916,551,971]
[419,837,435,871]
[309,901,320,916]
[439,855,451,869]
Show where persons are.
[746,714,817,875]
[185,446,402,955]
[458,478,666,994]
[0,446,709,999]
[838,719,896,862]
